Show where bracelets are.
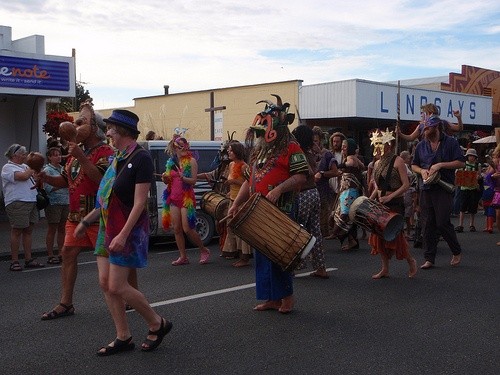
[80,219,89,228]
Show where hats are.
[103,108,141,133]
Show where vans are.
[141,140,258,246]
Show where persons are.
[161,136,211,265]
[0,144,69,272]
[291,103,500,279]
[73,109,173,357]
[228,102,309,313]
[31,103,138,323]
[197,139,254,267]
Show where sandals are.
[24,259,44,268]
[10,262,22,271]
[95,335,136,356]
[139,316,172,351]
[46,256,62,264]
[40,303,75,320]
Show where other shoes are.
[372,265,390,279]
[253,302,278,311]
[469,225,475,231]
[280,299,296,312]
[324,234,338,240]
[420,259,433,269]
[172,257,190,265]
[449,252,464,265]
[232,259,251,267]
[407,263,416,278]
[482,228,493,233]
[199,247,210,265]
[342,241,360,250]
[309,271,329,278]
[454,226,463,232]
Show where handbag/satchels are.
[37,192,49,211]
